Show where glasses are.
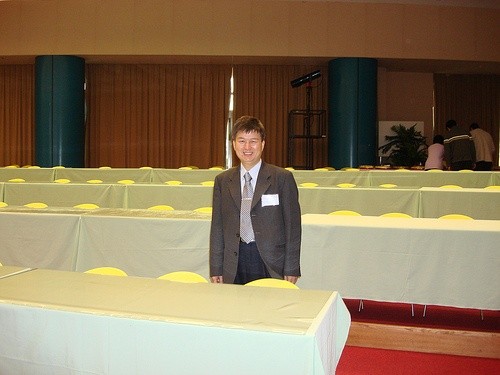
[236,139,259,148]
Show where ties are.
[240,173,253,245]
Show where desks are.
[0,182,500,221]
[0,263,351,375]
[0,167,500,188]
[0,205,500,312]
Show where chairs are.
[0,165,500,320]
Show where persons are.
[209,115,301,285]
[469,123,495,171]
[444,119,476,171]
[424,135,445,171]
